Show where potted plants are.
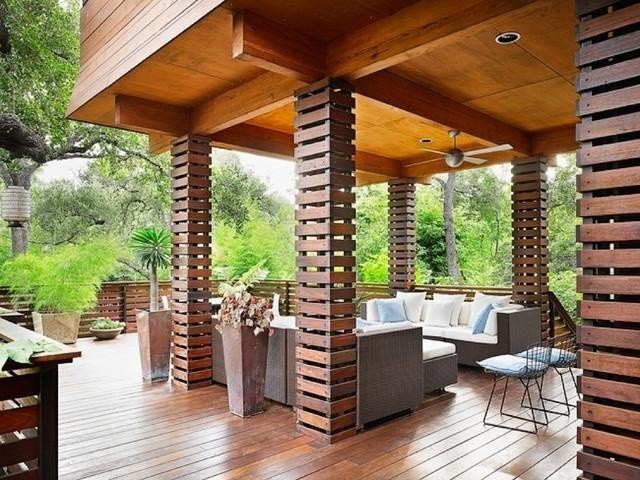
[90,317,126,340]
[0,233,132,344]
[130,227,172,384]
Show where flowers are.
[215,255,275,337]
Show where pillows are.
[376,290,511,334]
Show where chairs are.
[481,339,554,434]
[513,334,581,416]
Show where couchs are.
[361,298,541,367]
[211,315,423,432]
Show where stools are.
[423,339,458,395]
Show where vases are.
[220,323,270,418]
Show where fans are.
[402,129,513,168]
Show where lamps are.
[2,185,31,227]
